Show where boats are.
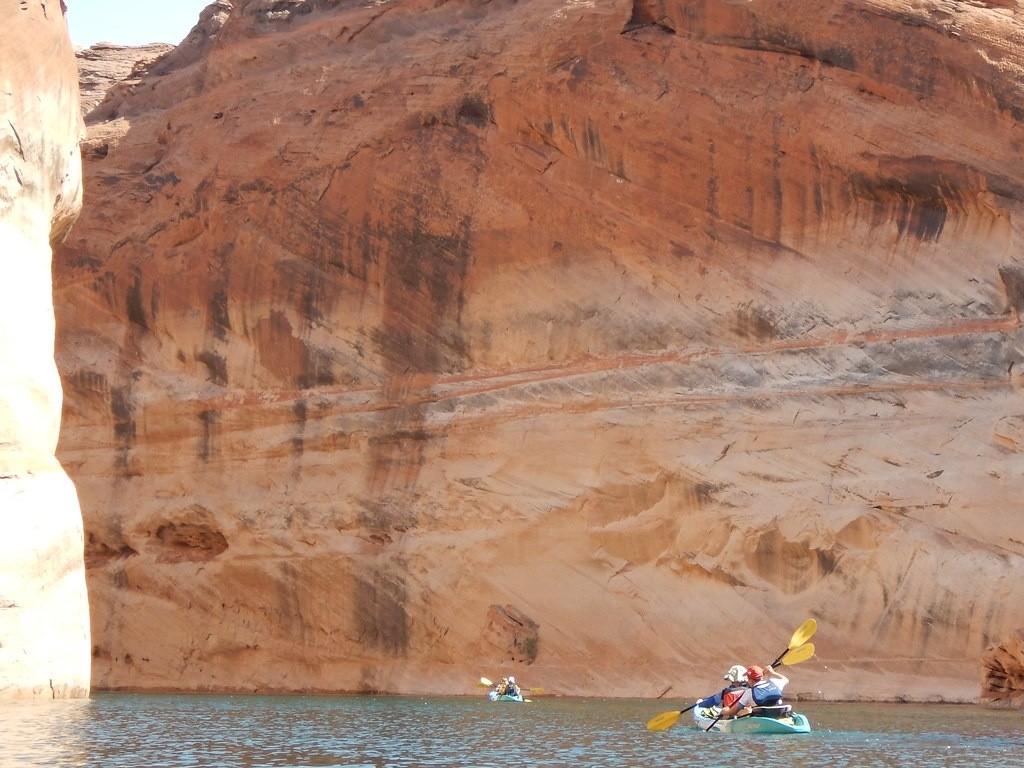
[692,704,811,735]
[490,687,525,703]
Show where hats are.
[724,665,750,683]
[743,665,763,680]
[508,676,515,683]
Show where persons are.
[721,665,789,718]
[696,665,752,717]
[494,677,520,696]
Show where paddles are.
[646,642,816,730]
[481,676,496,688]
[520,687,544,693]
[705,618,817,730]
